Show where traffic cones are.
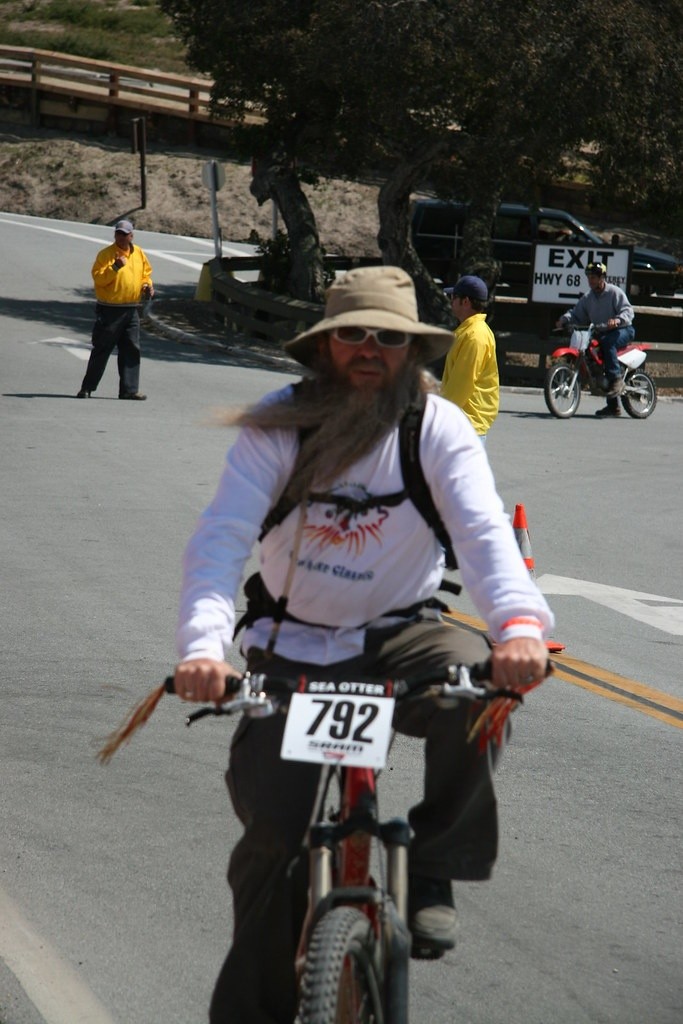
[511,502,534,579]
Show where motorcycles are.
[546,320,657,419]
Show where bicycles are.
[159,659,560,1024]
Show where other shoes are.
[596,404,621,417]
[409,876,459,949]
[77,389,90,398]
[607,378,626,398]
[118,391,146,400]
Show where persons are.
[556,261,635,418]
[434,274,500,450]
[173,265,557,1024]
[77,220,154,400]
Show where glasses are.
[332,327,413,348]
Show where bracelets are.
[503,618,543,629]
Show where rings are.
[184,692,193,697]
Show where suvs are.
[376,198,683,302]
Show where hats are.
[585,262,607,276]
[284,266,454,373]
[444,275,488,301]
[115,220,133,234]
